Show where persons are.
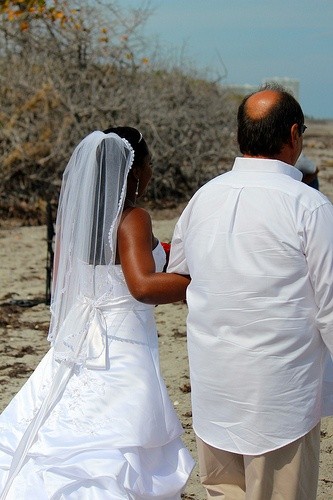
[0,126,192,500]
[166,82,333,500]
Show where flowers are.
[159,241,172,257]
